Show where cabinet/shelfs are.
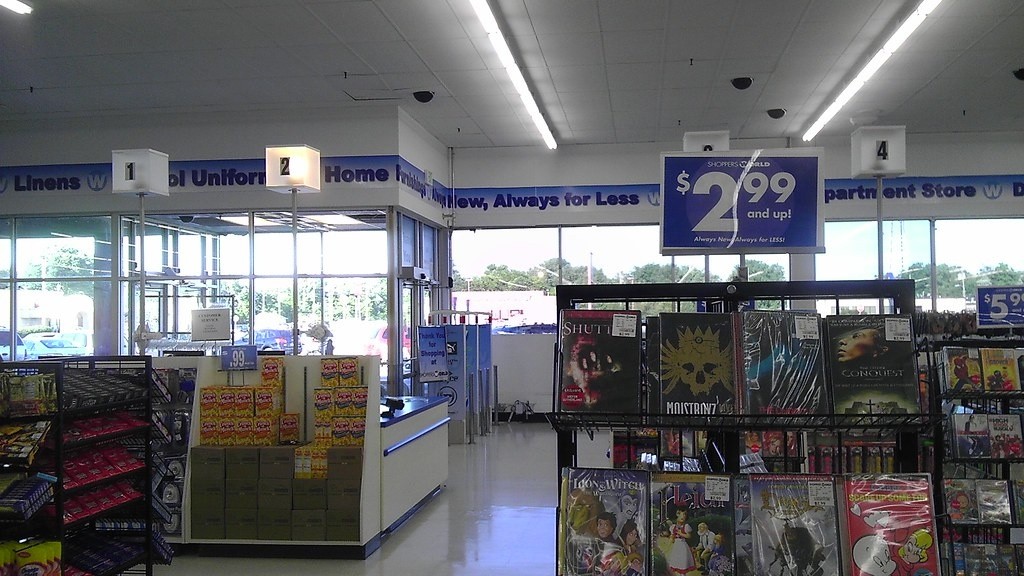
[0,354,153,576]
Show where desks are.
[380,394,453,541]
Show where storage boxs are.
[191,357,367,542]
[0,540,61,576]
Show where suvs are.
[236,328,301,355]
[0,326,27,362]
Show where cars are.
[22,332,87,359]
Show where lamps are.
[263,144,320,355]
[111,148,171,354]
[682,128,730,283]
[851,125,907,315]
